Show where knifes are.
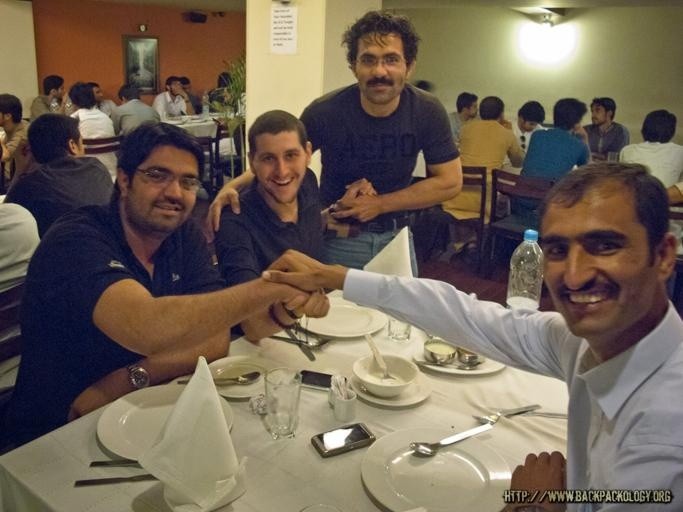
[488,405,569,422]
[73,474,160,488]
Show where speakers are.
[191,13,206,23]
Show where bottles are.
[64,96,74,116]
[200,91,209,119]
[505,230,544,312]
[48,98,61,115]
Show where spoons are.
[176,370,261,387]
[408,421,494,456]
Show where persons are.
[0,202,42,394]
[208,11,463,277]
[214,109,377,343]
[5,120,328,448]
[262,161,683,512]
[0,72,233,175]
[2,113,115,238]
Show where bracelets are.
[500,505,548,512]
[329,204,342,220]
[282,302,303,319]
[268,304,294,329]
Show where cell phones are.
[294,371,346,391]
[311,422,374,457]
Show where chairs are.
[0,115,682,510]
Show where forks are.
[471,403,541,422]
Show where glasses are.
[127,163,202,192]
[520,134,526,149]
[352,56,410,65]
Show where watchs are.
[126,362,150,388]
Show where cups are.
[261,366,303,437]
[607,151,618,164]
[329,376,358,423]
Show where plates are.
[209,300,505,408]
[160,112,221,126]
[93,384,234,461]
[361,424,513,512]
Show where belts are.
[336,215,412,235]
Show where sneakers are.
[460,240,480,268]
